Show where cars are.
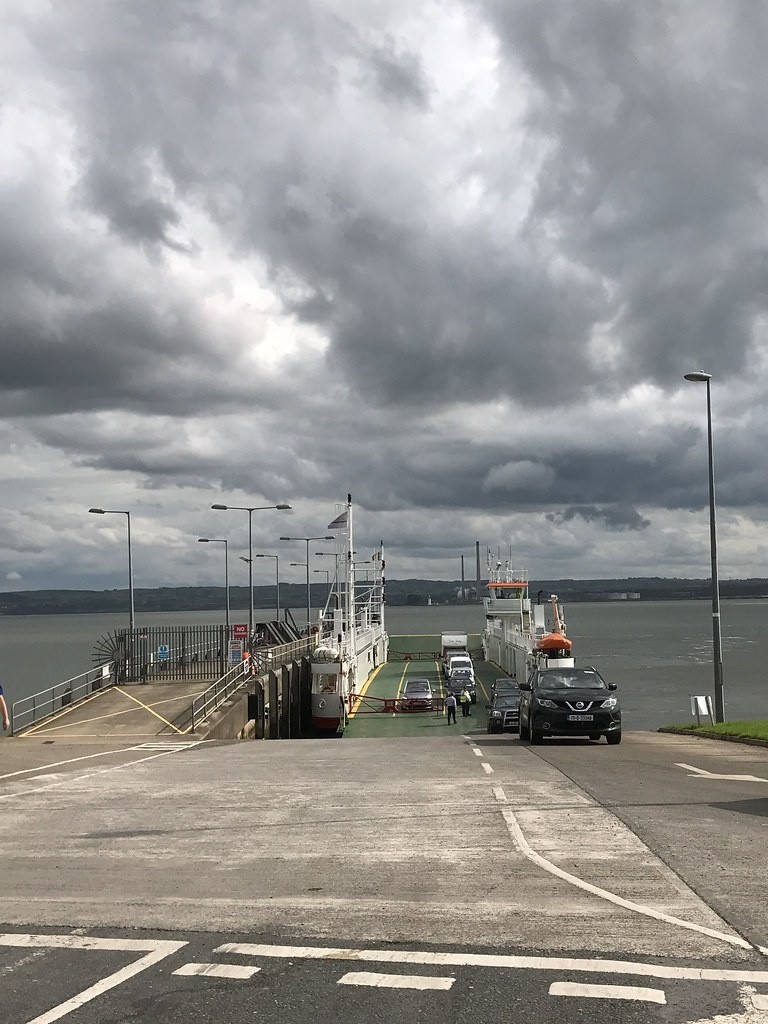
[443,649,476,686]
[491,679,521,703]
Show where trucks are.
[440,630,468,676]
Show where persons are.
[461,687,472,717]
[444,693,458,725]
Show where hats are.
[451,692,455,696]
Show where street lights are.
[683,370,725,726]
[255,554,280,627]
[353,560,371,597]
[314,569,330,628]
[315,552,340,610]
[279,534,336,654]
[197,538,231,641]
[89,507,135,630]
[210,504,293,644]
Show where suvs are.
[518,665,622,745]
[444,675,477,706]
[399,677,436,710]
[485,694,522,734]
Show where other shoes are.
[454,722,457,724]
[467,714,471,716]
[464,716,467,717]
[448,724,450,725]
